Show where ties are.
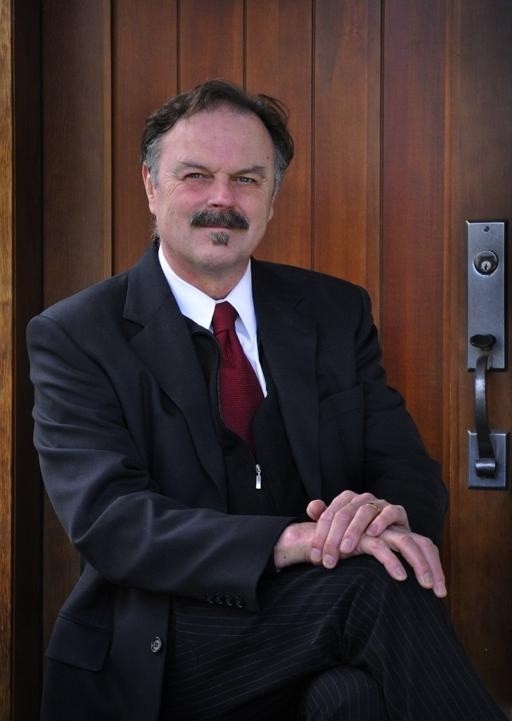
[212,301,265,463]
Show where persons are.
[26,78,457,721]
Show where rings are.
[366,500,381,514]
